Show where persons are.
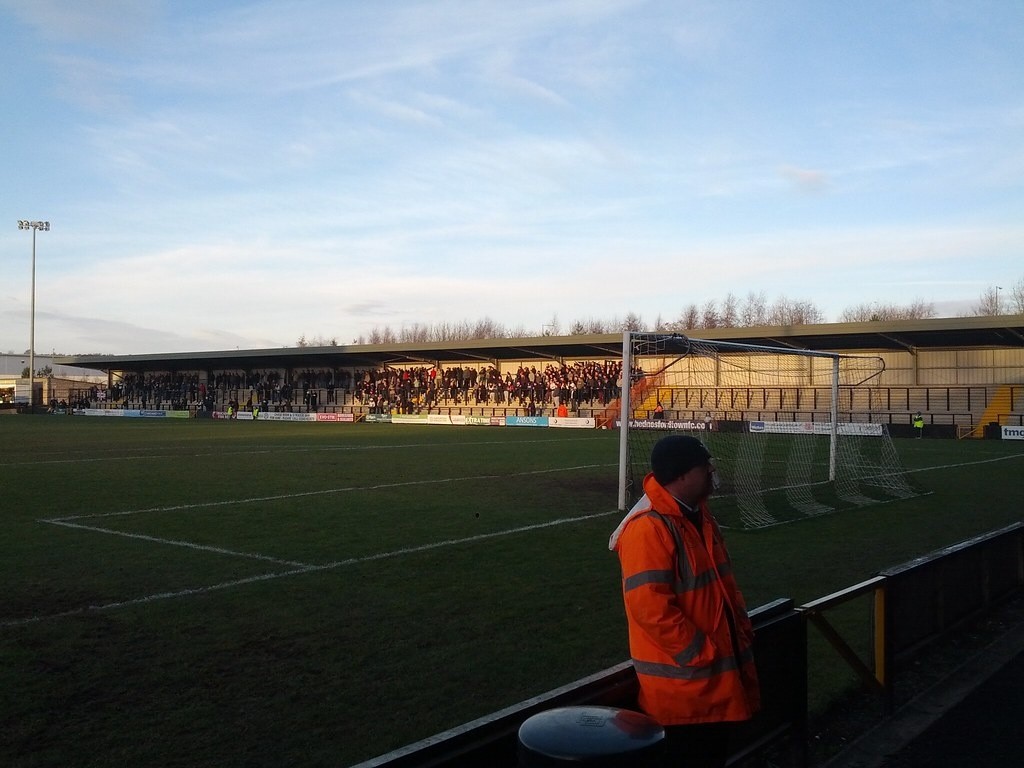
[653,401,664,419]
[704,411,713,432]
[607,434,763,768]
[913,410,924,440]
[0,360,645,420]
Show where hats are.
[652,436,712,486]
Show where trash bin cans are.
[518,707,666,768]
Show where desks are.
[517,703,667,768]
